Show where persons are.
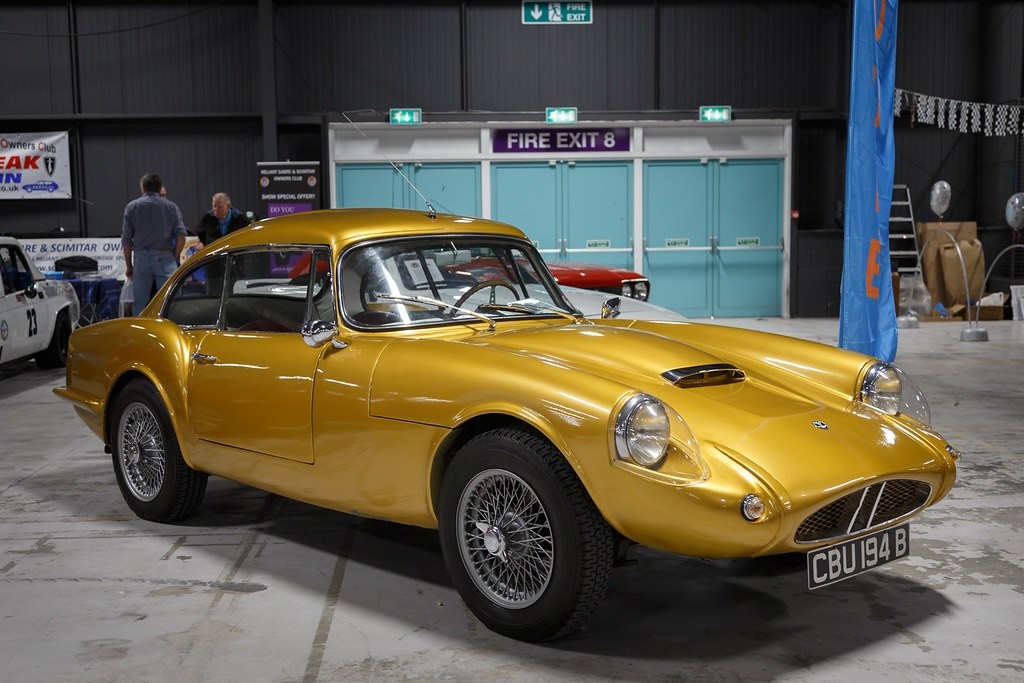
[196,193,246,298]
[122,174,187,316]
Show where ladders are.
[889,183,925,290]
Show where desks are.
[48,278,122,328]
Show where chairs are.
[311,256,393,321]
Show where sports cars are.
[50,207,961,644]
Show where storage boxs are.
[916,221,1011,322]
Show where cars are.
[0,236,81,370]
[233,250,687,323]
[289,246,651,304]
